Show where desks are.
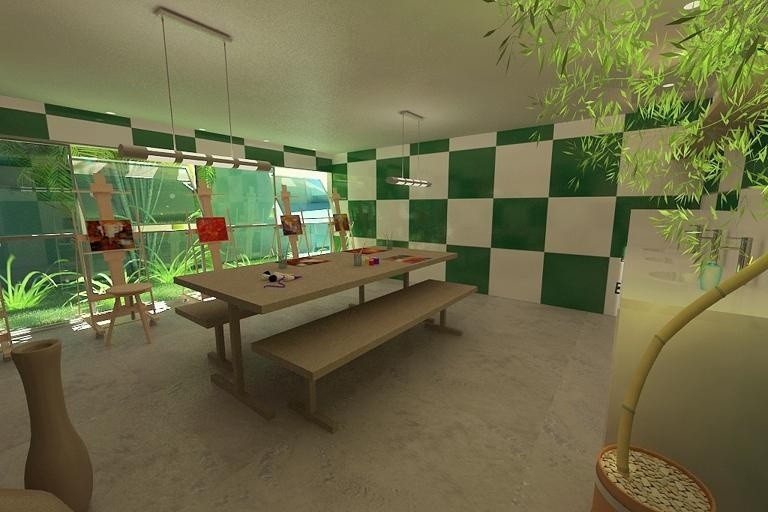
[174,246,458,419]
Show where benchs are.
[175,286,365,372]
[250,280,478,433]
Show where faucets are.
[696,227,722,265]
[683,224,706,252]
[719,234,753,272]
[676,227,682,248]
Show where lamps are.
[118,8,272,172]
[387,110,431,187]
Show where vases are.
[10,339,93,512]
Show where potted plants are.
[483,0,768,512]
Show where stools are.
[105,284,156,345]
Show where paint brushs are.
[359,241,365,256]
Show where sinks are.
[643,247,671,252]
[651,270,699,285]
[646,258,677,264]
